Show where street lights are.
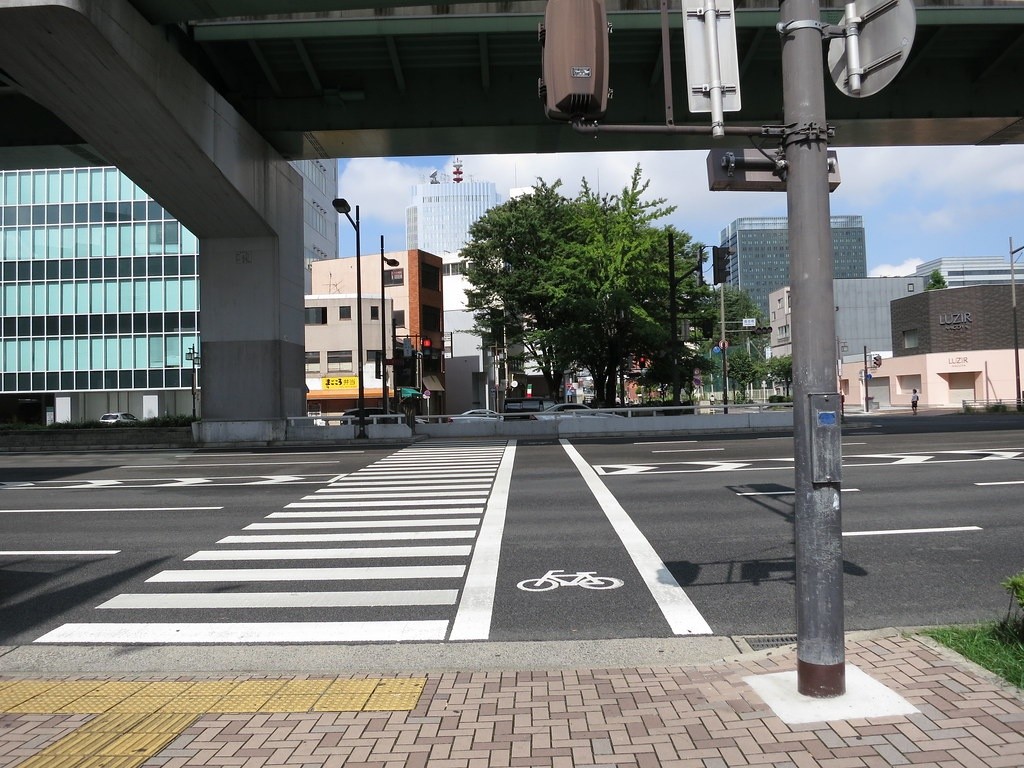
[380,257,400,423]
[186,347,201,418]
[332,197,370,440]
[488,346,507,412]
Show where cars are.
[530,402,625,419]
[340,408,424,424]
[99,412,140,424]
[449,409,505,422]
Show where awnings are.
[307,388,397,398]
[402,388,422,399]
[423,376,445,392]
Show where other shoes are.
[913,413,917,415]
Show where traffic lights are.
[873,355,882,366]
[713,247,733,287]
[423,338,432,361]
[753,326,773,335]
[639,357,646,368]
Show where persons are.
[710,395,715,405]
[911,388,919,416]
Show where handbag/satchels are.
[916,396,919,401]
